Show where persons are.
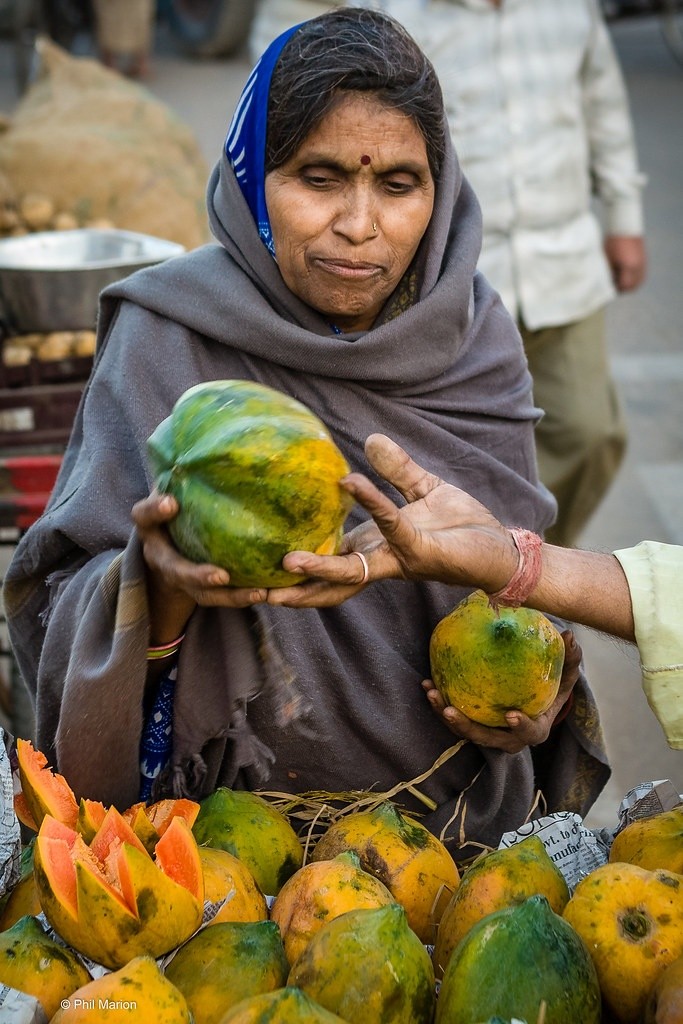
[247,0,646,547]
[266,434,683,751]
[0,9,611,851]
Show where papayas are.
[429,585,566,728]
[145,379,357,590]
[0,736,683,1024]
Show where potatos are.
[0,192,115,241]
[1,327,97,367]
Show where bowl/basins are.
[0,228,187,338]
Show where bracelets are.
[147,633,185,660]
[494,529,541,605]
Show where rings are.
[348,552,367,585]
